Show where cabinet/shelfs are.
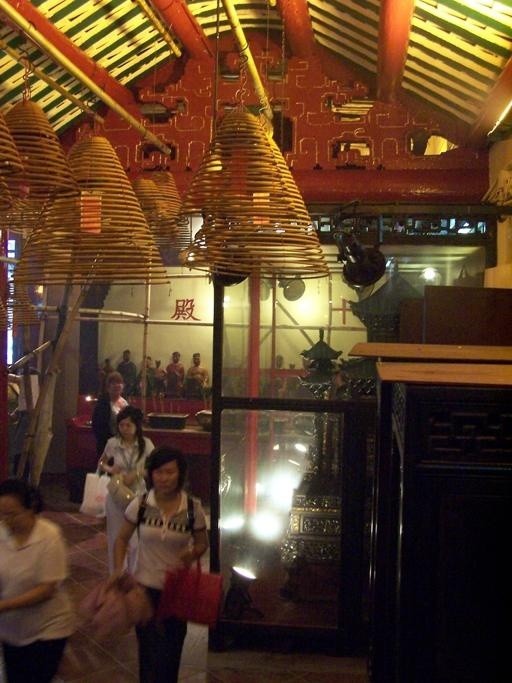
[368,380,511,683]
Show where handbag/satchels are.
[79,461,110,519]
[106,471,135,510]
[156,549,222,625]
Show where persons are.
[96,349,208,400]
[1,479,76,683]
[114,445,207,682]
[8,364,41,476]
[97,404,157,574]
[93,370,130,470]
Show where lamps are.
[9,114,172,289]
[2,45,84,237]
[170,43,333,280]
[121,124,197,269]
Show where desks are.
[66,413,212,503]
